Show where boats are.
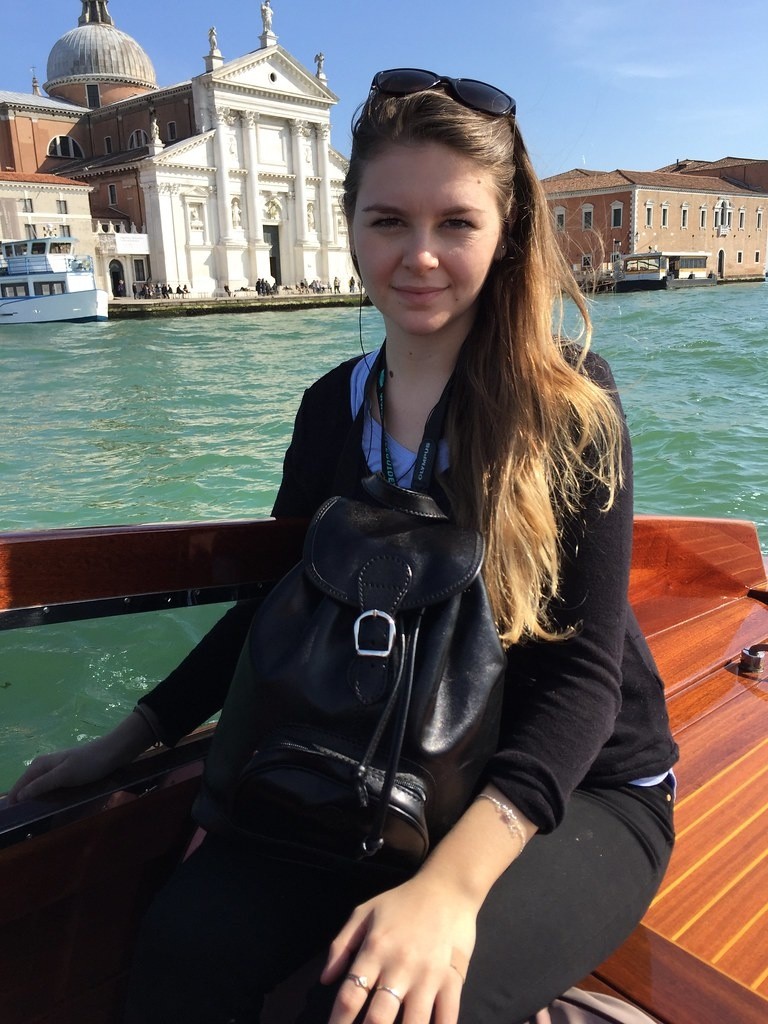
[613,245,719,292]
[0,235,112,324]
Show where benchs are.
[136,287,331,300]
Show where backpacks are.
[180,486,520,881]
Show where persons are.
[132,280,191,300]
[295,278,309,294]
[224,283,231,297]
[118,279,126,296]
[6,68,679,1024]
[311,279,326,294]
[349,276,355,293]
[239,287,250,291]
[627,264,649,272]
[334,277,341,294]
[327,281,333,294]
[255,278,278,297]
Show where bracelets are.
[478,794,526,853]
[133,705,160,748]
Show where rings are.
[376,987,403,1003]
[347,973,373,991]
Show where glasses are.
[367,67,519,143]
